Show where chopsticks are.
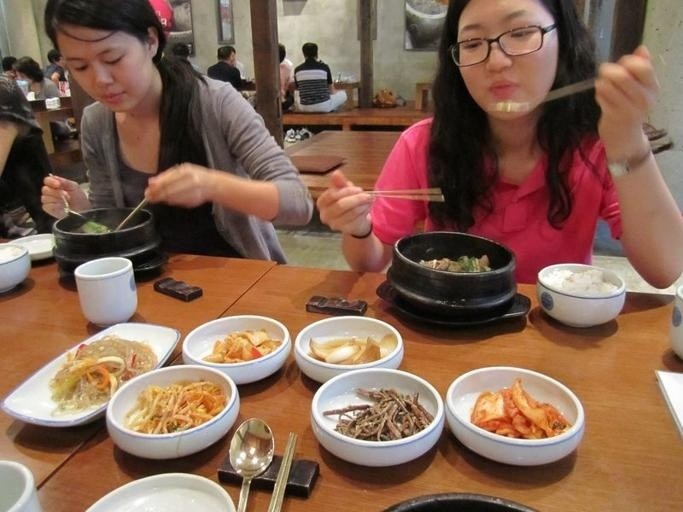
[313,188,445,202]
[267,432,299,512]
[114,199,148,233]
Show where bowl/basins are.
[311,368,445,469]
[182,315,292,385]
[536,263,626,328]
[294,314,404,381]
[445,366,585,468]
[0,244,30,294]
[106,365,240,459]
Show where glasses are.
[447,23,559,67]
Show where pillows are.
[290,155,347,174]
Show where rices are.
[542,268,620,299]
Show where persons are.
[1,55,70,242]
[40,1,313,266]
[207,46,257,92]
[279,43,348,113]
[171,43,191,58]
[317,0,683,290]
[43,49,67,82]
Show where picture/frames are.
[215,0,235,46]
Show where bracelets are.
[339,219,378,240]
[605,144,652,179]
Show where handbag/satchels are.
[372,88,406,108]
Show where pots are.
[53,207,164,264]
[386,232,518,310]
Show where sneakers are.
[285,128,297,143]
[295,127,314,140]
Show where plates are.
[375,280,531,324]
[57,254,170,272]
[84,472,237,512]
[0,322,180,427]
[8,232,55,261]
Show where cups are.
[1,456,44,512]
[74,256,138,327]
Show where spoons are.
[49,173,86,220]
[229,417,275,512]
